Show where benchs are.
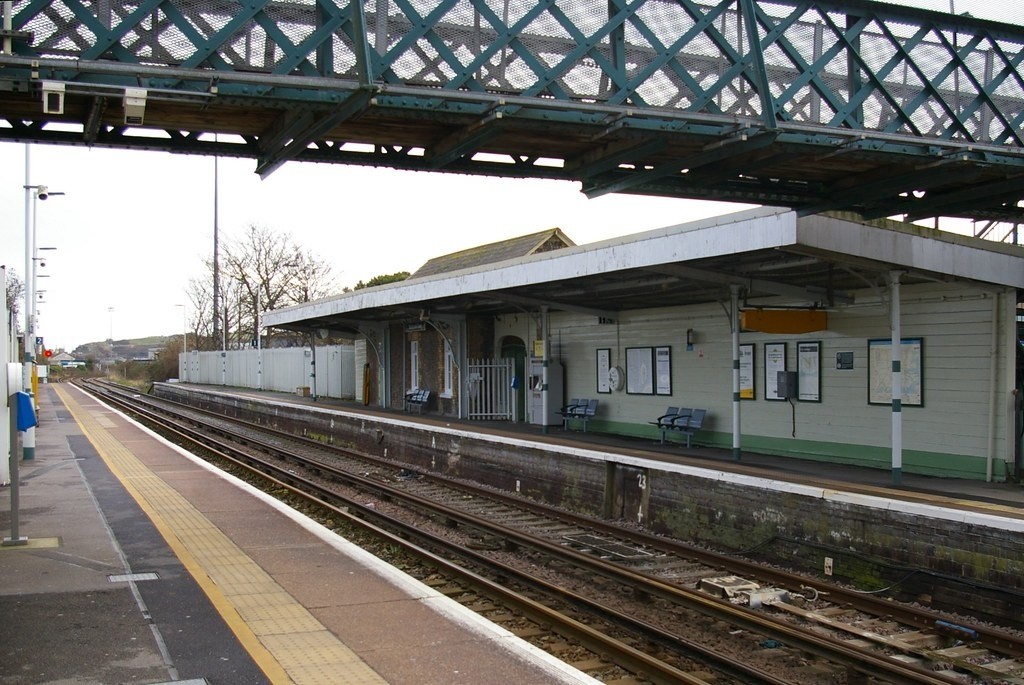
[554,398,599,432]
[649,407,707,448]
[402,388,431,415]
